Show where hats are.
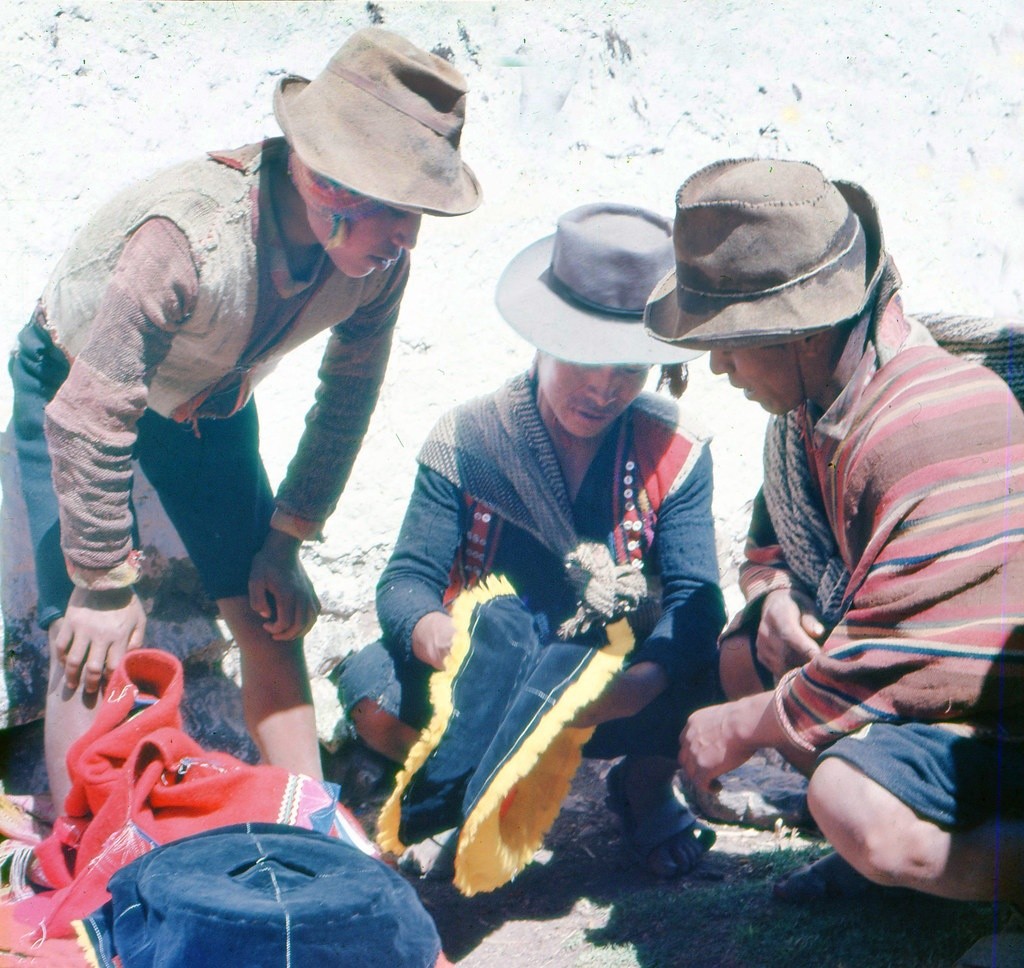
[492,201,708,369]
[641,154,886,350]
[271,27,483,217]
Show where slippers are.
[604,763,716,878]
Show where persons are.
[9,23,482,823]
[642,159,1024,903]
[332,203,727,880]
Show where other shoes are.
[776,852,921,906]
